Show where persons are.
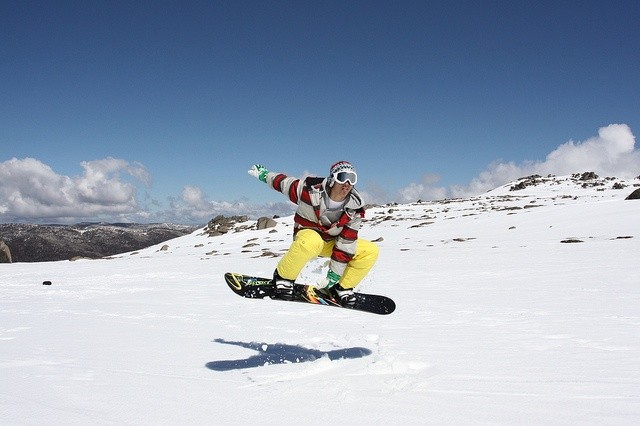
[248,161,380,306]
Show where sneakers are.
[329,283,356,306]
[273,268,295,298]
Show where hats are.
[329,161,355,188]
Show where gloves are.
[248,163,270,183]
[324,269,340,290]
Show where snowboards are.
[224,272,396,316]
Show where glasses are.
[331,170,358,185]
